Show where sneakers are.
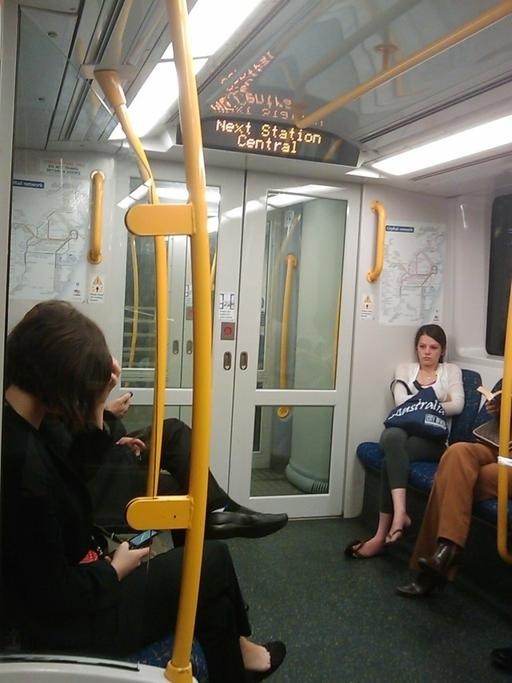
[244,639,286,683]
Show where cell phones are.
[125,392,133,403]
[107,530,163,560]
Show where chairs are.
[114,630,208,683]
[356,369,512,537]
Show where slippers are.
[345,536,383,559]
[385,524,413,545]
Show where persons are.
[342,321,466,561]
[393,380,512,596]
[1,295,289,683]
[48,383,289,621]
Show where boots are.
[417,540,457,582]
[395,569,451,598]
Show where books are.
[474,384,501,403]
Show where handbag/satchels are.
[384,385,449,443]
[471,416,512,452]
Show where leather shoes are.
[206,502,288,540]
